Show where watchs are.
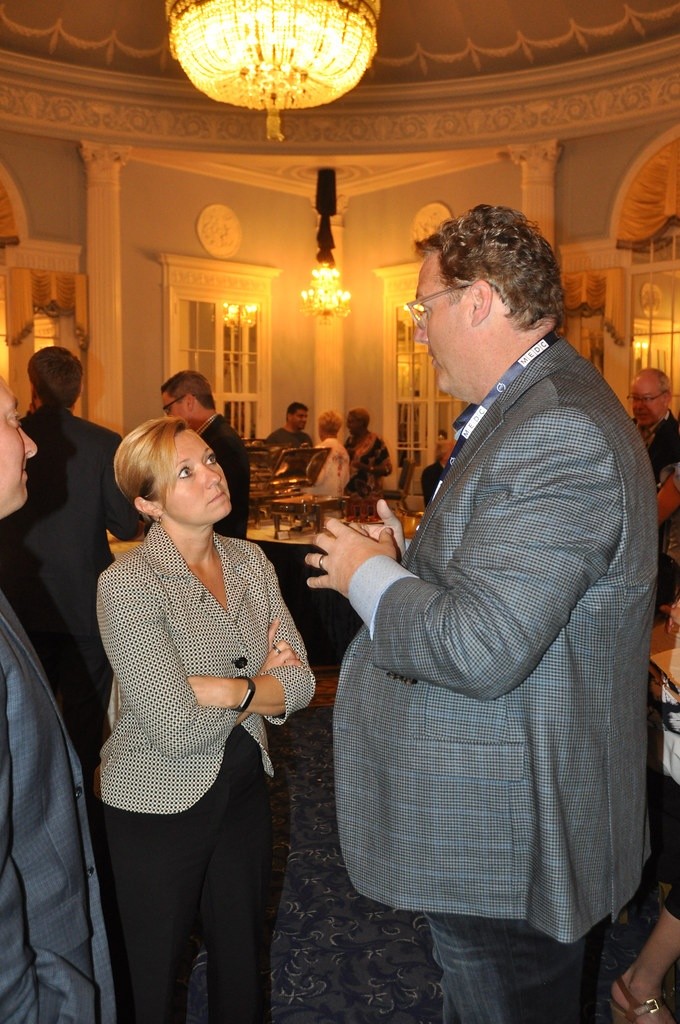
[231,676,255,712]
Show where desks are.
[246,518,415,669]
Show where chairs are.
[383,456,416,515]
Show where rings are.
[273,646,281,654]
[319,555,325,570]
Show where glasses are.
[163,393,196,415]
[406,282,506,330]
[626,389,668,404]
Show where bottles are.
[341,486,384,522]
[310,495,323,533]
[300,503,316,538]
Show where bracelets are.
[369,467,374,472]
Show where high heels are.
[610,976,664,1024]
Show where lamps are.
[301,168,353,324]
[210,303,257,339]
[164,0,382,142]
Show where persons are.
[306,411,350,495]
[0,345,140,808]
[627,368,680,614]
[160,370,251,540]
[98,415,316,1024]
[267,402,313,447]
[304,204,658,1024]
[344,407,393,498]
[608,881,680,1024]
[0,376,116,1024]
[421,439,456,508]
